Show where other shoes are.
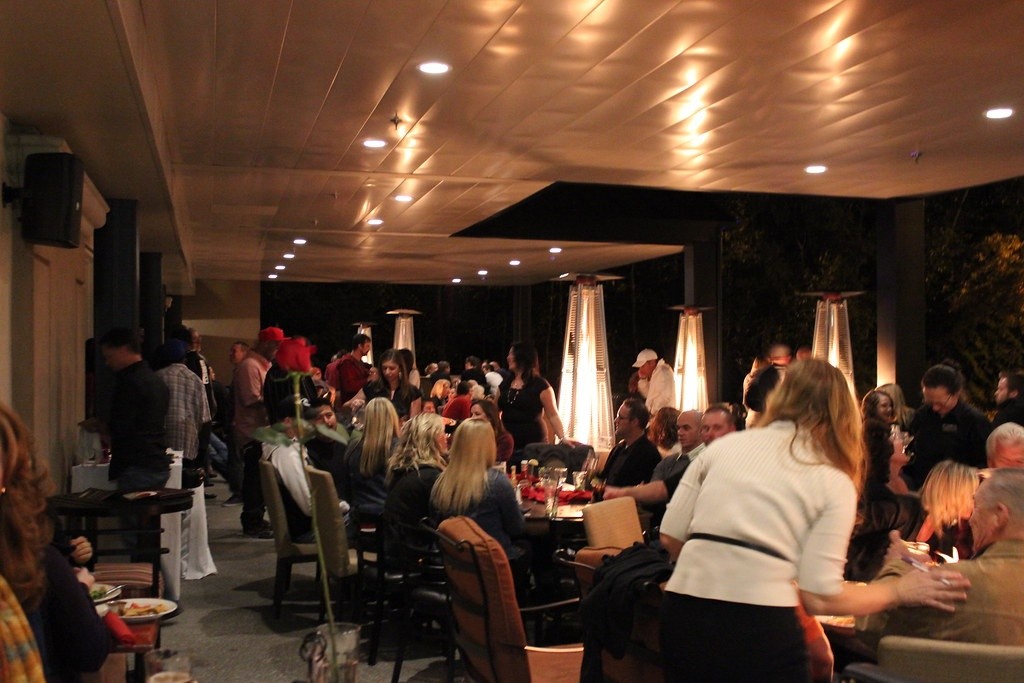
[208,474,217,478]
[222,496,244,506]
[242,524,274,538]
[205,483,215,487]
[262,520,272,528]
[205,494,217,500]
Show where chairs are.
[259,441,666,683]
[64,529,169,649]
[877,636,1024,683]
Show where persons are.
[742,345,812,427]
[843,365,1024,649]
[659,358,970,683]
[78,327,228,620]
[226,326,745,640]
[0,403,110,683]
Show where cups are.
[144,647,192,683]
[538,467,588,519]
[310,623,360,683]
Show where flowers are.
[247,326,350,681]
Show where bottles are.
[500,452,533,517]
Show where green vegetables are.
[90,590,106,599]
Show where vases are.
[311,623,362,683]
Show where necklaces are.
[515,380,517,385]
[508,383,523,404]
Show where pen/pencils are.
[901,556,951,587]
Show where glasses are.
[617,413,633,421]
[921,393,954,410]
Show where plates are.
[94,596,178,622]
[90,583,121,602]
[123,491,158,499]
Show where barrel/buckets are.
[75,426,103,463]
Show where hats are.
[258,327,291,342]
[632,348,658,367]
[166,339,186,363]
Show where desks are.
[46,488,193,566]
[506,473,592,536]
[71,451,184,599]
